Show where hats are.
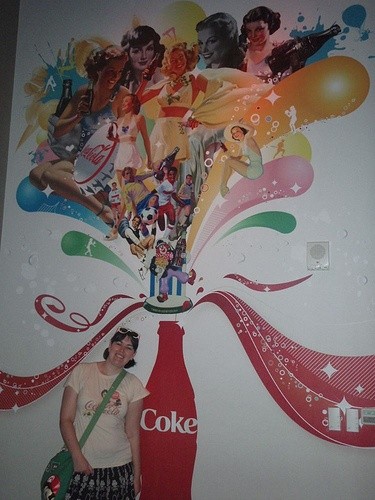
[45,476,61,495]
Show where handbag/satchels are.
[40,450,73,500]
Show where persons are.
[59,327,151,500]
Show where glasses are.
[116,327,140,342]
